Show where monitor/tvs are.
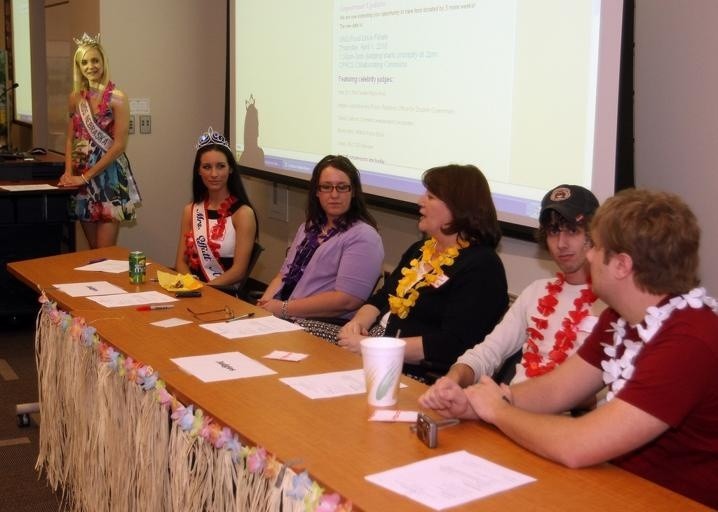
[0,49,13,154]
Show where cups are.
[360,336,407,408]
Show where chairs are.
[370,270,390,297]
[168,243,266,300]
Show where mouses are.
[30,147,47,155]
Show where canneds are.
[129,250,146,285]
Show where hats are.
[539,184,600,226]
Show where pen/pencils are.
[136,304,174,311]
[226,313,255,324]
[88,258,105,264]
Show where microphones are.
[0,83,18,98]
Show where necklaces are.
[427,241,459,267]
[319,216,339,236]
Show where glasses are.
[187,304,234,322]
[318,185,352,193]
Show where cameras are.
[416,414,438,448]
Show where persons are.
[445,183,621,421]
[258,155,384,349]
[417,187,718,509]
[175,127,258,299]
[336,162,511,394]
[58,31,143,249]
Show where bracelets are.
[81,173,89,185]
[281,300,289,320]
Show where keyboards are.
[1,154,24,159]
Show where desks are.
[1,180,80,317]
[7,247,718,512]
